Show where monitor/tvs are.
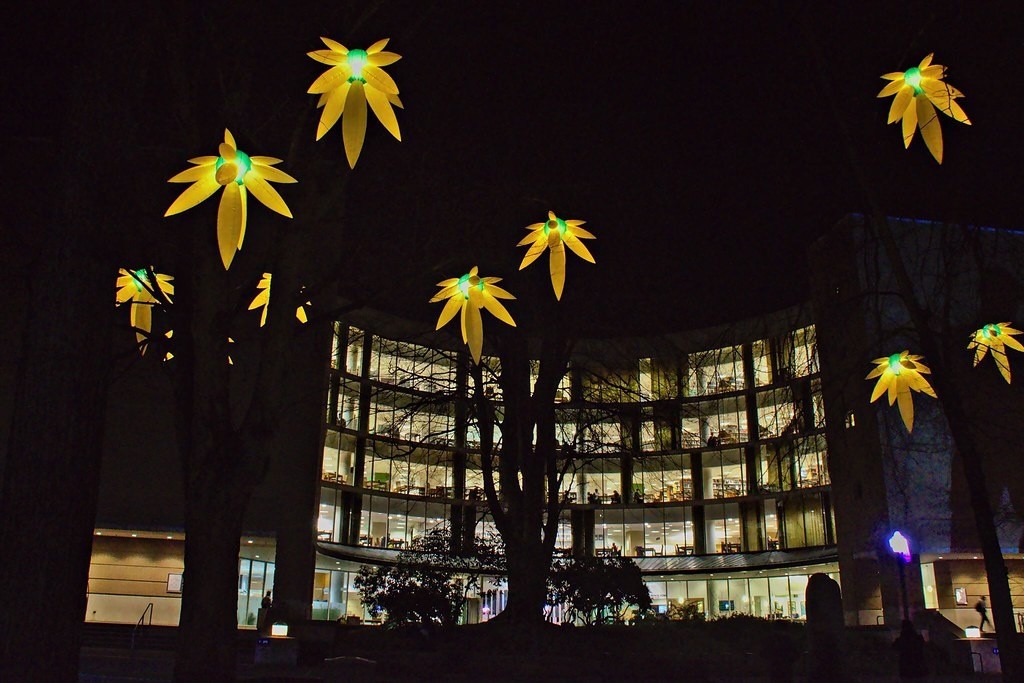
[166,573,182,593]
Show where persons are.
[337,414,346,427]
[611,490,619,502]
[975,596,994,632]
[892,620,929,683]
[611,543,617,556]
[564,490,570,499]
[708,433,717,446]
[593,489,601,504]
[634,489,645,503]
[759,619,800,683]
[261,592,272,608]
